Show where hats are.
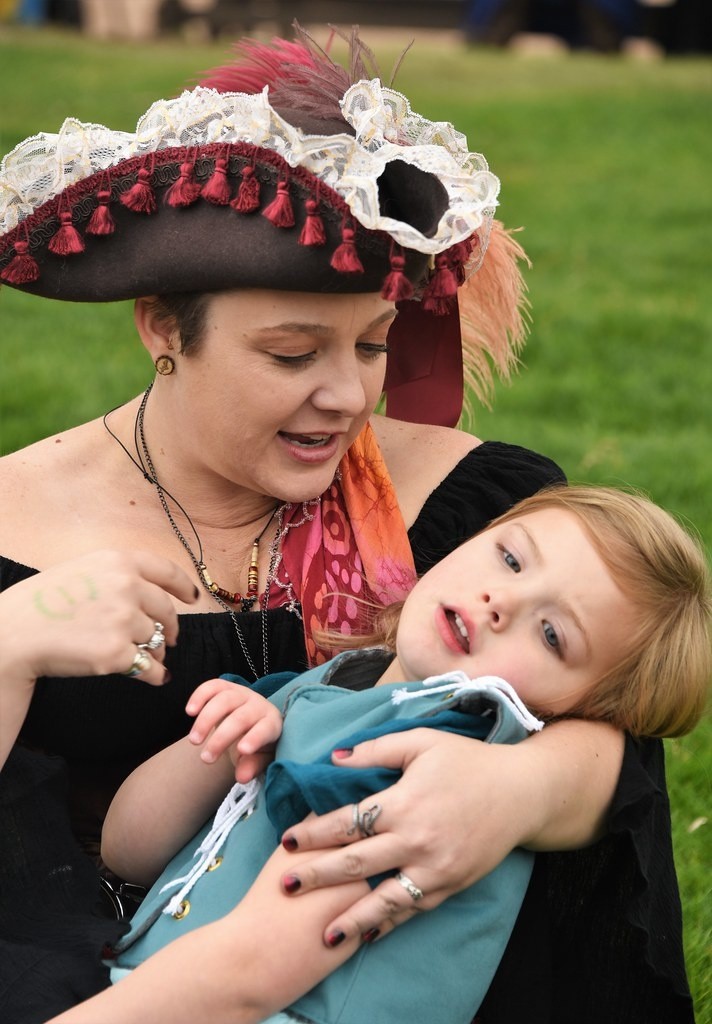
[0,20,532,431]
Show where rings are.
[138,622,165,652]
[118,650,151,679]
[396,870,423,906]
[346,801,381,838]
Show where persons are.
[44,484,711,1024]
[0,24,696,1023]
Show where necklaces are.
[104,382,283,679]
